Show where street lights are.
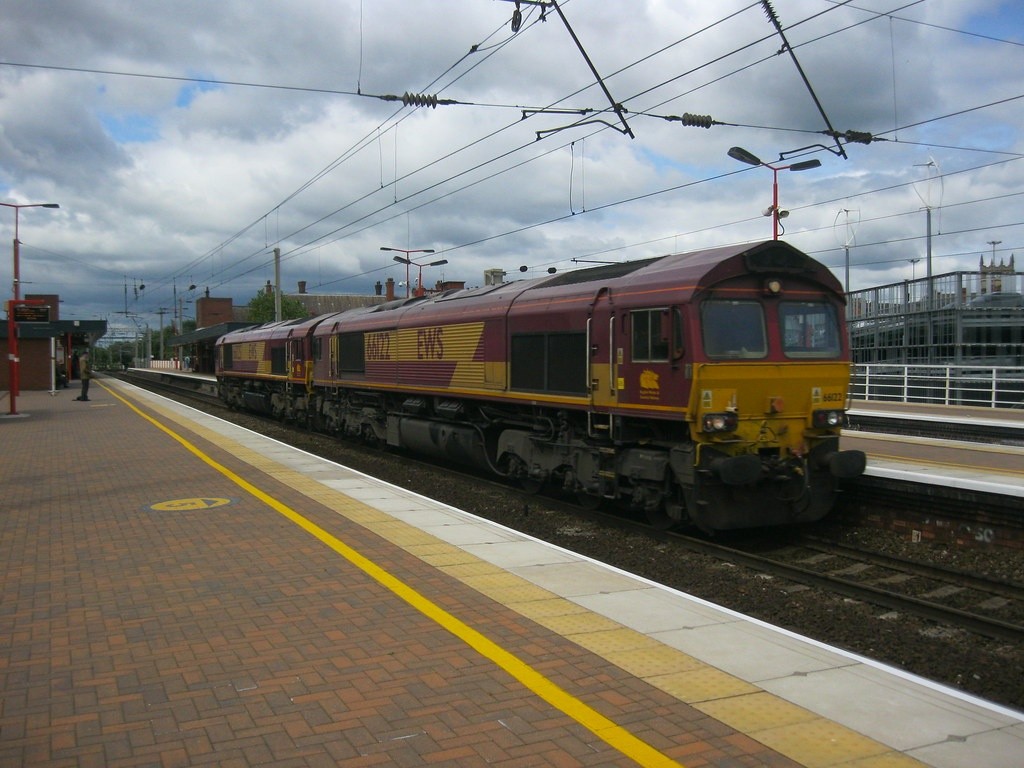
[0,203,61,415]
[393,256,448,297]
[726,146,823,242]
[379,247,435,298]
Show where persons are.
[79,352,92,401]
[185,357,190,370]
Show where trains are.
[215,238,866,540]
